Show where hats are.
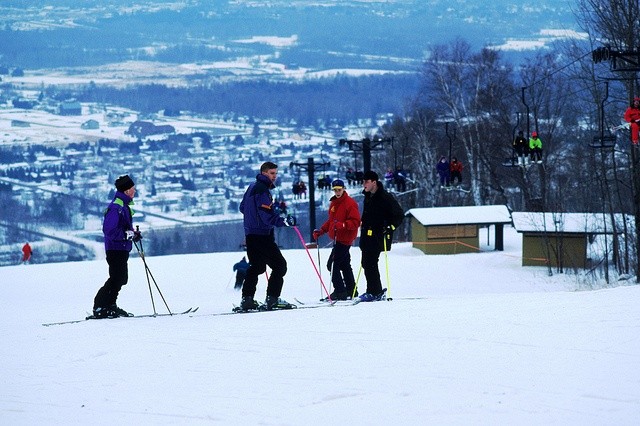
[532,131,537,137]
[115,175,135,192]
[360,171,378,179]
[332,180,345,191]
[633,98,640,100]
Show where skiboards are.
[41,307,199,327]
[291,296,360,307]
[353,294,427,301]
[230,298,338,315]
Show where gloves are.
[125,230,142,242]
[331,221,345,229]
[382,224,394,241]
[283,215,295,227]
[313,229,324,240]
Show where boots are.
[518,156,523,166]
[524,156,529,165]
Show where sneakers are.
[536,160,542,164]
[109,305,127,316]
[359,293,367,297]
[530,161,535,163]
[93,307,115,316]
[363,288,387,300]
[265,295,291,308]
[320,292,347,301]
[346,289,357,297]
[240,297,264,310]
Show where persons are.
[449,157,464,188]
[513,131,531,166]
[625,97,640,146]
[360,171,405,302]
[317,174,332,190]
[292,181,307,199]
[233,257,250,289]
[528,131,543,163]
[394,166,406,192]
[239,162,300,310]
[22,242,33,264]
[346,168,355,187]
[313,180,361,302]
[437,158,452,188]
[355,168,364,186]
[93,174,139,318]
[384,168,395,193]
